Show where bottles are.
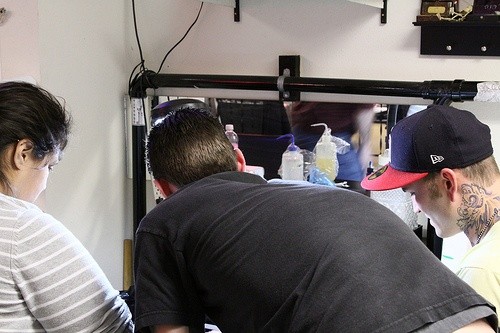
[224,124,238,149]
[316,136,336,182]
[282,145,303,181]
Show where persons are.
[283,100,380,199]
[135,107,500,333]
[0,81,135,333]
[361,105,500,330]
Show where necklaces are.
[473,209,500,245]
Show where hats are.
[360,101,494,193]
